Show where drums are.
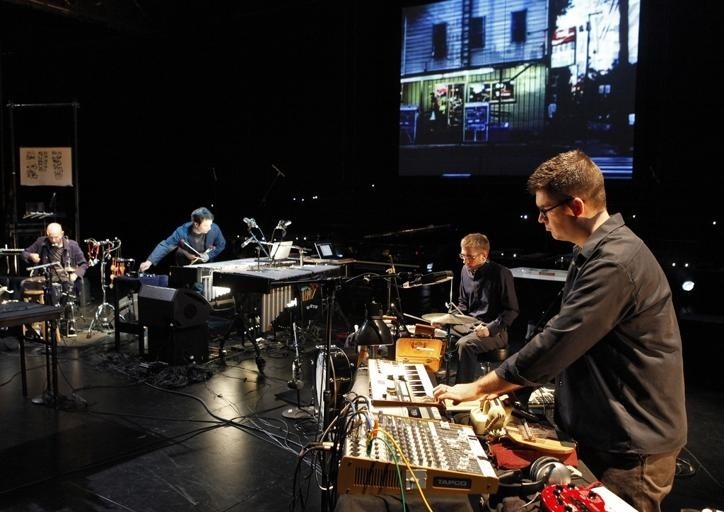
[314,348,351,408]
[372,316,397,337]
[52,282,77,305]
[399,325,449,339]
[20,277,46,293]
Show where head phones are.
[496,455,571,496]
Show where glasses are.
[534,200,571,217]
[459,253,483,260]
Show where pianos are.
[368,358,443,405]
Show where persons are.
[454,233,519,384]
[22,222,88,295]
[139,207,225,271]
[434,150,688,512]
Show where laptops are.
[254,241,293,262]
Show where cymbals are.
[403,275,455,290]
[27,263,59,270]
[422,314,477,325]
[57,267,78,272]
[0,249,24,254]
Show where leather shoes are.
[68,321,75,334]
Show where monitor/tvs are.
[314,242,336,260]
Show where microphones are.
[421,272,447,284]
[276,219,292,229]
[241,236,255,248]
[42,243,64,249]
[243,217,258,229]
[288,358,304,389]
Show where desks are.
[511,265,570,298]
[183,256,349,372]
[336,409,605,512]
[0,299,68,410]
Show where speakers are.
[137,285,214,364]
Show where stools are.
[477,348,507,374]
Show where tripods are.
[86,245,126,339]
[247,246,270,271]
